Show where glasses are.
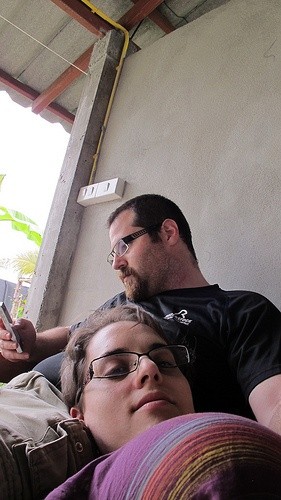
[106,226,163,266]
[74,344,191,406]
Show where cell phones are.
[0,302,24,354]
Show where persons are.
[0,194,281,500]
[46,305,281,499]
